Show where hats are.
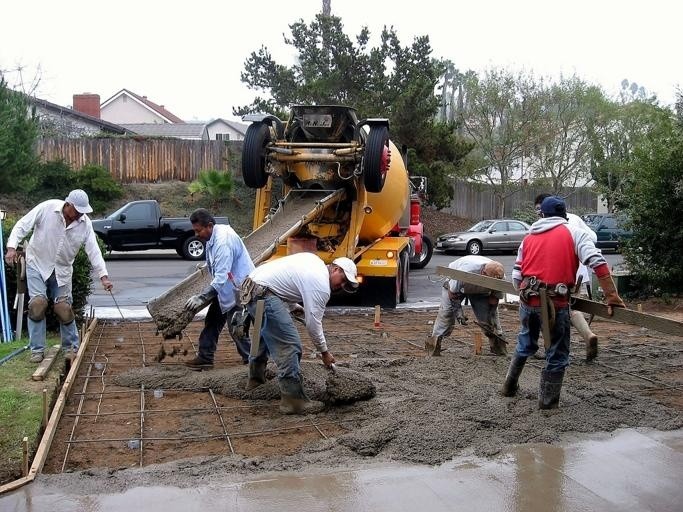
[66,189,94,214]
[539,196,566,217]
[332,257,358,284]
[484,260,505,279]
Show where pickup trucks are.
[91,200,231,262]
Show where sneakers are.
[30,351,45,363]
[184,356,214,369]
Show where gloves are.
[450,297,468,325]
[597,272,626,315]
[185,284,219,311]
[487,303,498,331]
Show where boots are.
[571,310,599,362]
[279,376,325,415]
[244,360,269,391]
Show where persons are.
[245,252,359,416]
[4,188,114,363]
[180,208,255,371]
[500,196,628,409]
[424,254,510,357]
[534,194,598,361]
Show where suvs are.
[579,213,636,255]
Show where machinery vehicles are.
[142,103,437,350]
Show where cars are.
[434,217,530,257]
